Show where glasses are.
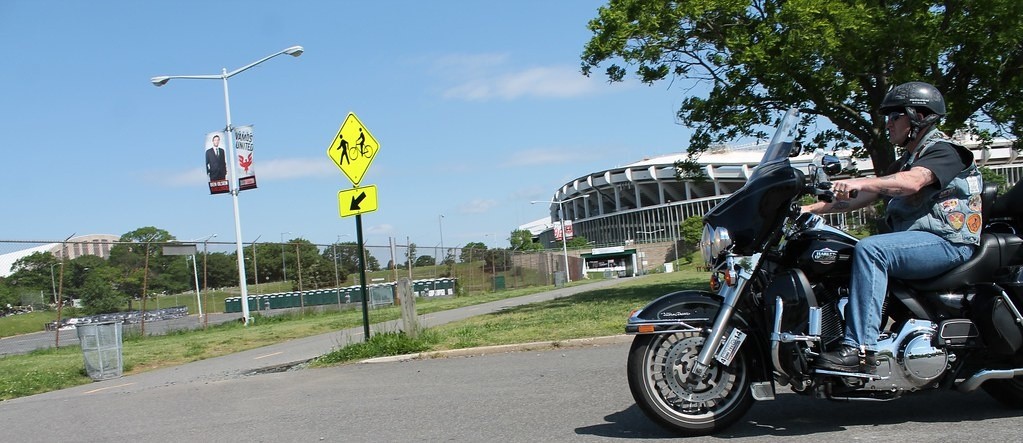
[889,112,907,120]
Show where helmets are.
[879,81,946,117]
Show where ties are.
[216,148,219,156]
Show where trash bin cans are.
[224,277,459,312]
[75,319,124,381]
[491,276,505,293]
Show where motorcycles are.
[623,106,1023,438]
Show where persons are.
[206,135,227,180]
[795,82,984,372]
[345,292,351,304]
[264,296,270,312]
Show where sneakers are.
[811,346,877,374]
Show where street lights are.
[439,215,445,259]
[281,232,292,282]
[531,194,591,284]
[636,228,664,243]
[151,46,304,326]
[203,233,218,328]
[50,263,62,303]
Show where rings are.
[834,188,839,192]
[839,189,844,192]
